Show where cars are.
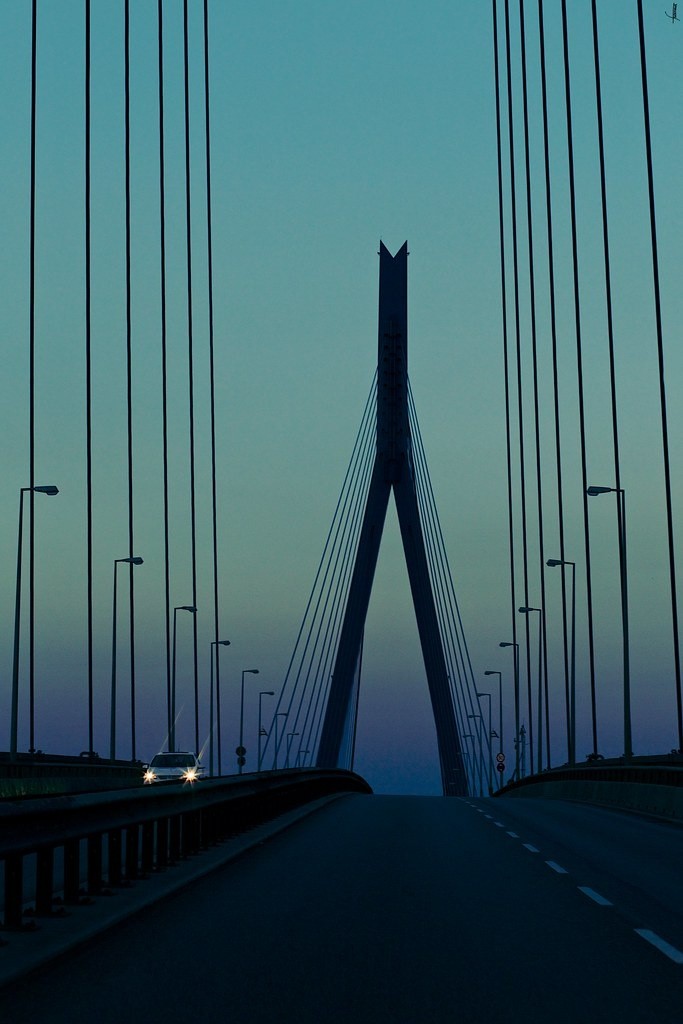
[141,752,206,785]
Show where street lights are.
[547,558,578,766]
[469,714,483,797]
[298,751,310,769]
[499,642,520,782]
[110,558,145,766]
[484,670,504,789]
[10,485,60,761]
[286,733,300,768]
[208,640,231,777]
[477,693,493,797]
[518,607,544,775]
[274,712,289,770]
[462,734,476,797]
[586,484,633,758]
[258,691,274,773]
[172,606,198,753]
[238,670,260,774]
